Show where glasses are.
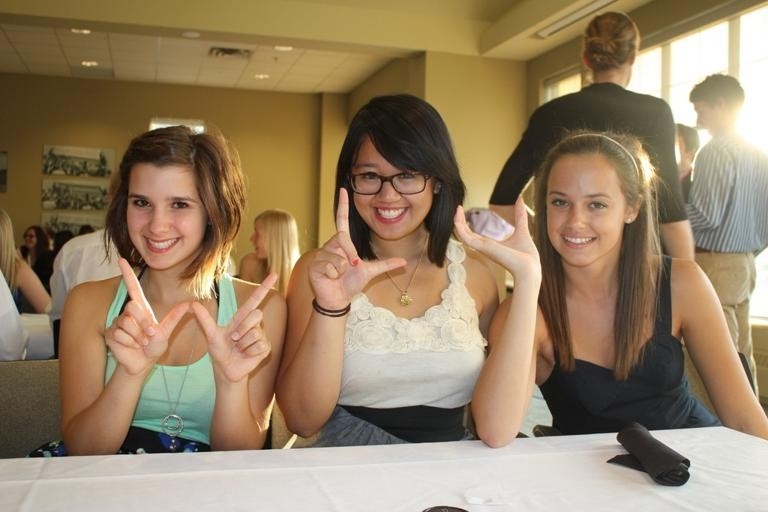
[350,172,434,195]
[23,233,36,238]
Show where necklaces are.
[369,231,430,305]
[161,298,208,437]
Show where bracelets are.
[312,298,351,317]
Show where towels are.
[606,420,691,487]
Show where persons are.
[49,227,122,358]
[274,94,499,448]
[25,126,287,459]
[39,230,73,296]
[0,207,52,315]
[673,123,699,203]
[16,225,49,273]
[683,74,767,418]
[489,11,695,259]
[238,211,300,299]
[79,225,93,235]
[453,132,768,448]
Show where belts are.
[696,247,711,253]
